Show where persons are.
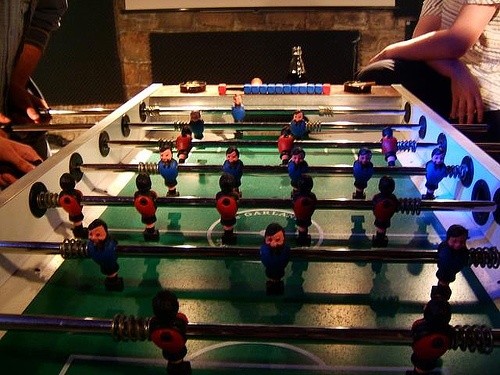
[358,0,500,128]
[0,0,69,187]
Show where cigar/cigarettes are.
[355,82,375,87]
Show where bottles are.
[287,47,307,84]
